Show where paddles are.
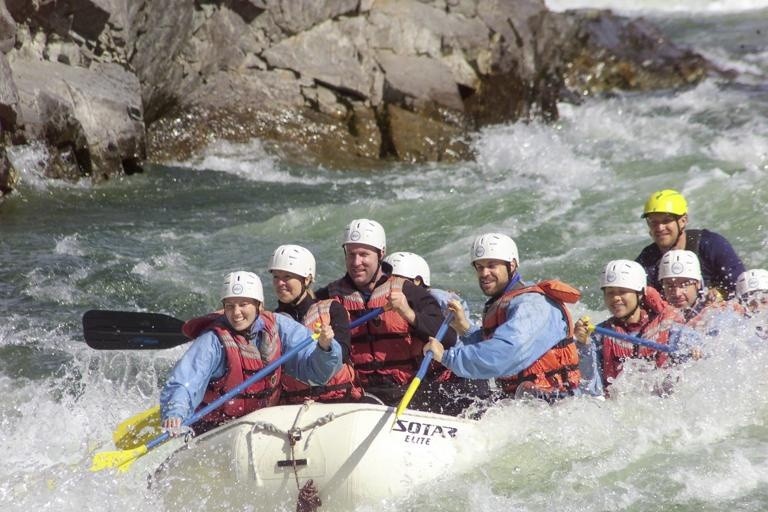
[392,304,455,428]
[49,321,325,490]
[81,309,195,350]
[115,301,396,453]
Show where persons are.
[268,246,365,404]
[422,232,580,422]
[575,261,701,400]
[382,252,469,321]
[314,219,456,413]
[635,190,768,324]
[159,271,343,429]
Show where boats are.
[146,404,485,511]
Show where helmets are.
[221,270,265,304]
[737,268,768,298]
[381,250,432,287]
[658,249,702,280]
[268,244,316,280]
[342,219,387,255]
[470,233,520,267]
[641,188,688,217]
[600,259,647,293]
